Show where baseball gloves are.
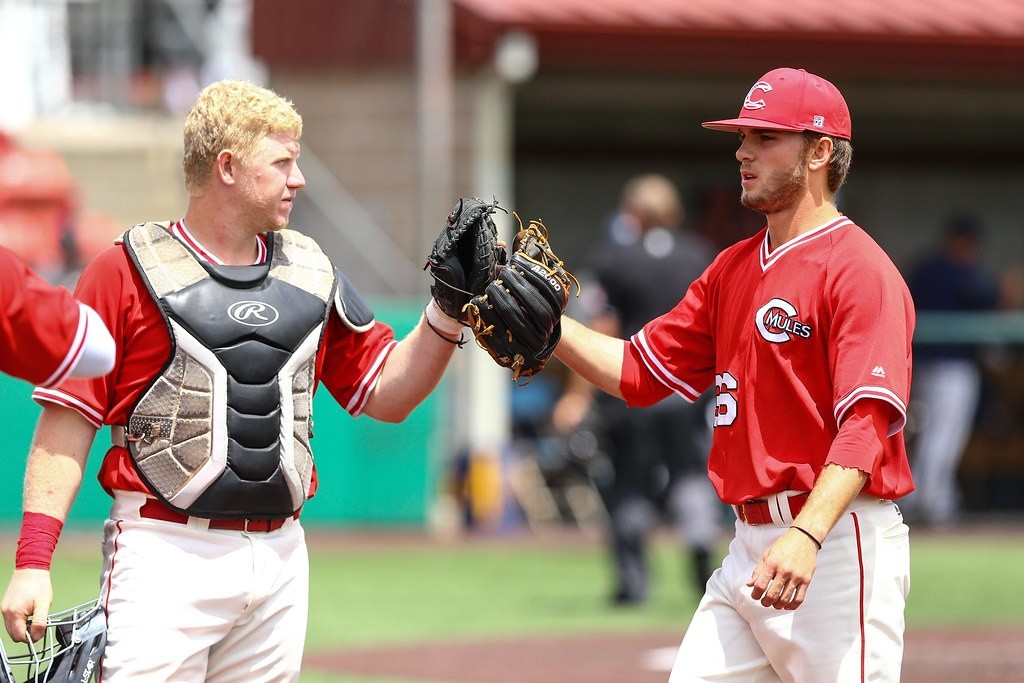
[428,195,508,320]
[467,224,572,378]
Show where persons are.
[548,172,1024,603]
[0,78,507,683]
[460,67,917,683]
[0,246,116,390]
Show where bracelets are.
[789,526,822,550]
[424,302,470,349]
[15,512,63,571]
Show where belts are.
[140,495,301,532]
[736,493,811,526]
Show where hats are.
[701,67,853,141]
